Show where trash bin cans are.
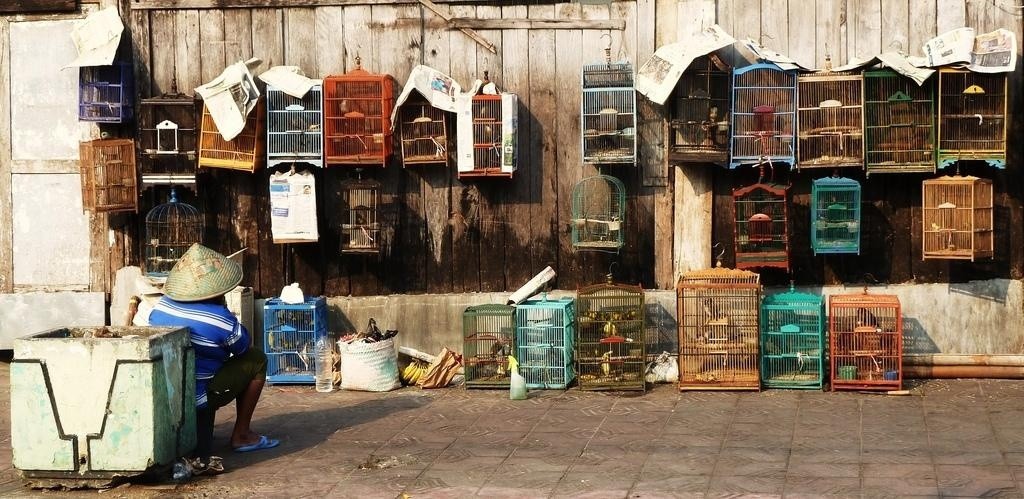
[9,324,200,490]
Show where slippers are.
[233,435,280,453]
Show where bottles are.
[315,328,333,393]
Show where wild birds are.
[704,298,720,322]
[857,307,880,328]
[880,327,895,347]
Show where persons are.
[146,241,281,453]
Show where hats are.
[160,242,243,304]
